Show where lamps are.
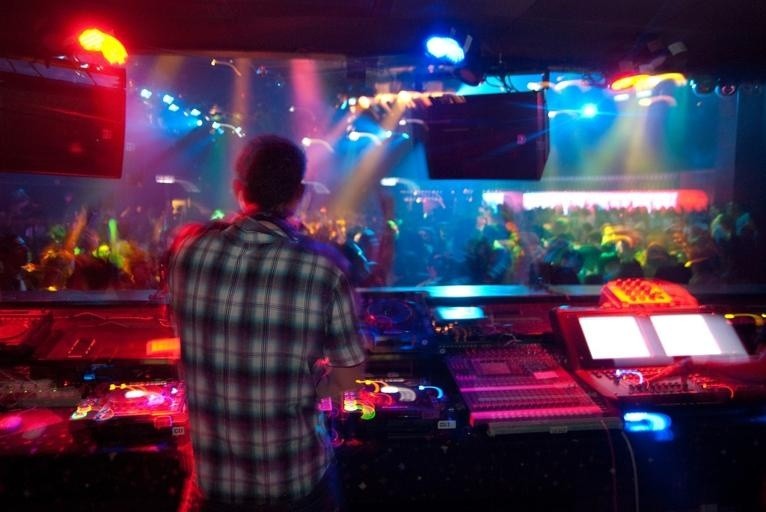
[424,22,477,66]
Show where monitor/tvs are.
[557,305,667,368]
[646,308,751,365]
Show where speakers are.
[423,90,550,181]
[0,51,126,179]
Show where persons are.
[167,133,368,511]
[0,197,233,291]
[301,186,765,289]
